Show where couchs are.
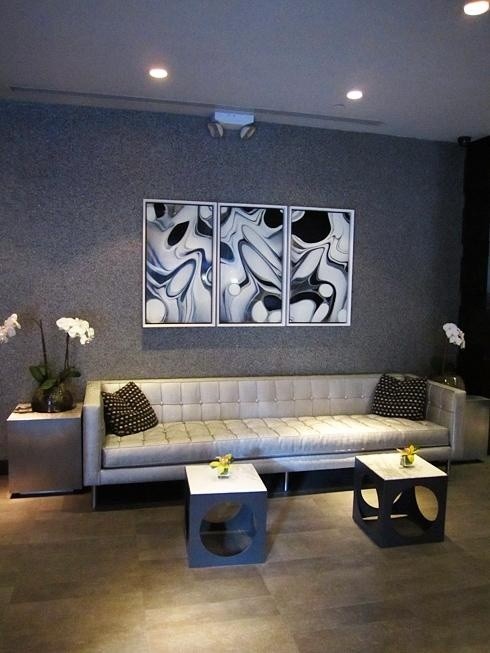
[84,373,467,510]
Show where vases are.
[32,388,73,413]
[434,375,466,393]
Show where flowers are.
[441,322,467,374]
[0,314,94,385]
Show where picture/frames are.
[218,203,287,328]
[140,198,217,330]
[286,204,355,327]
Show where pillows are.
[102,382,159,435]
[370,372,426,421]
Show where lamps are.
[240,126,256,139]
[207,122,225,140]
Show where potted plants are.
[211,454,233,478]
[396,444,420,468]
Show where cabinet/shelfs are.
[462,396,489,460]
[6,403,83,496]
